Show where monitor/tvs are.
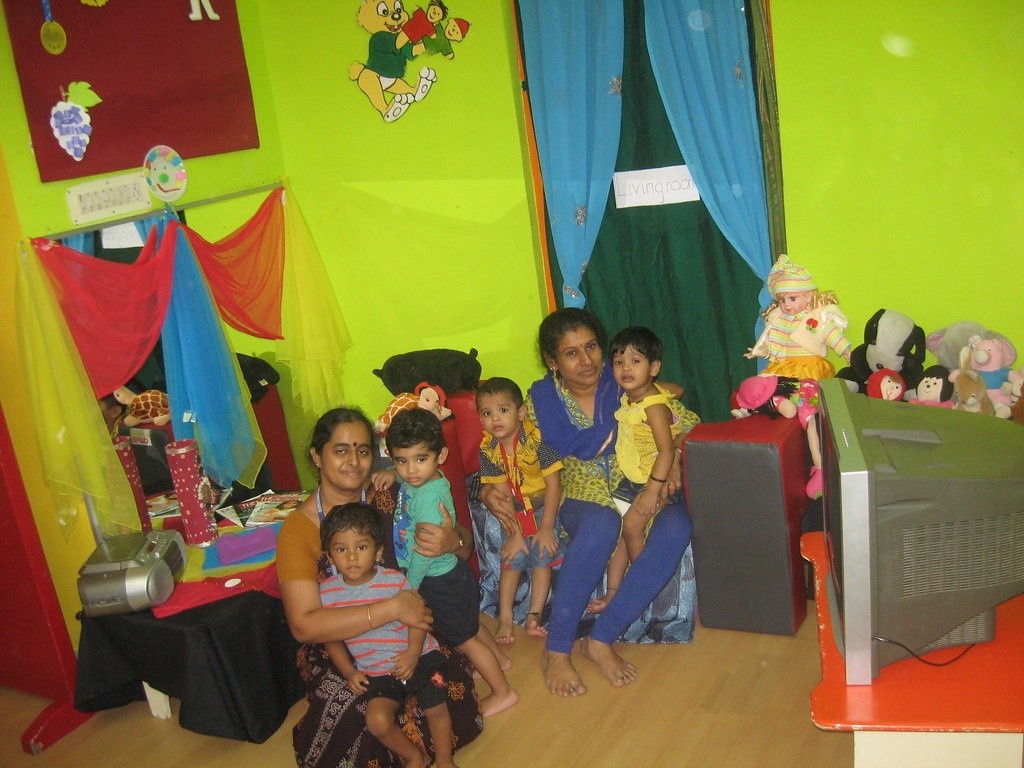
[819,377,1024,686]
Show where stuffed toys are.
[906,363,955,409]
[114,386,172,426]
[372,382,456,435]
[835,307,1024,426]
[866,368,906,402]
[736,375,822,499]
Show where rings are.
[669,482,674,485]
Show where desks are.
[800,531,1024,768]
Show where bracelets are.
[457,535,464,546]
[675,447,682,454]
[649,475,667,483]
[367,603,374,631]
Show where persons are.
[370,407,517,717]
[97,396,132,435]
[586,327,684,614]
[477,307,703,696]
[275,499,302,519]
[731,254,852,418]
[477,376,571,643]
[277,408,483,768]
[318,502,459,768]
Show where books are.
[216,488,309,530]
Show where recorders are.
[77,530,187,618]
